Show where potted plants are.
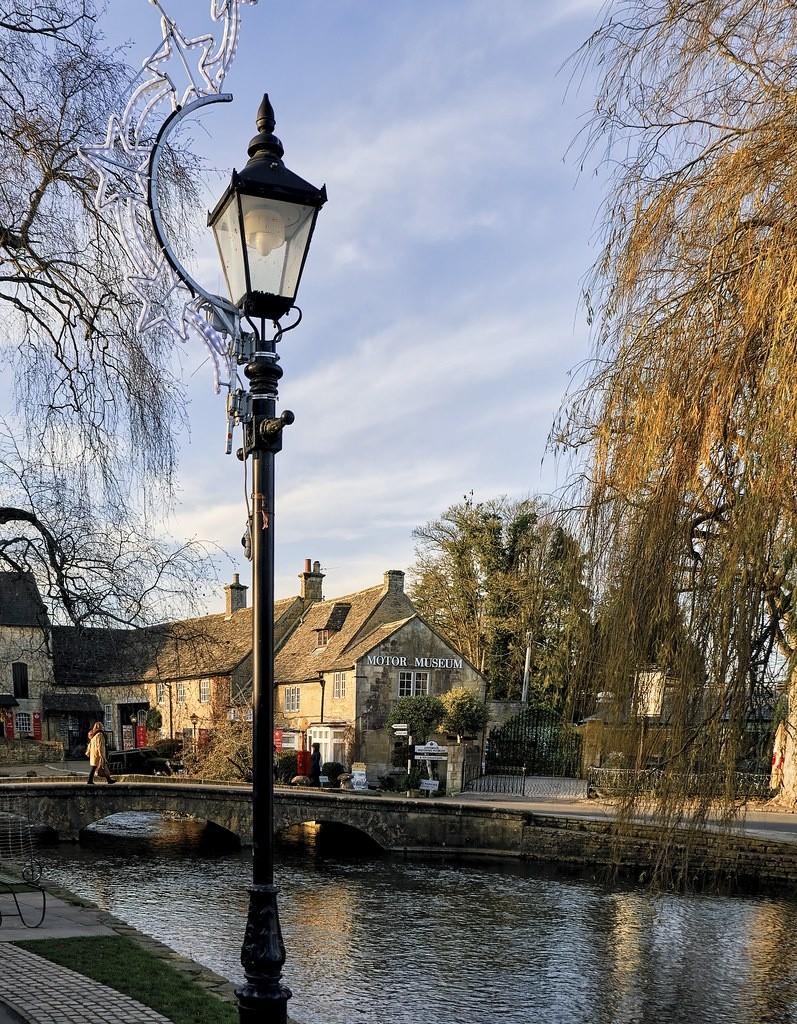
[400,768,421,797]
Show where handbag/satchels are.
[95,758,110,777]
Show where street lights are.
[205,98,328,1023]
[190,714,198,759]
[130,713,138,747]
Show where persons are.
[85,722,117,784]
[308,743,321,787]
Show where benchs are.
[0,858,46,928]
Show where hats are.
[311,743,316,749]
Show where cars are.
[108,747,184,776]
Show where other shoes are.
[108,779,117,784]
[88,781,95,784]
[311,782,321,787]
